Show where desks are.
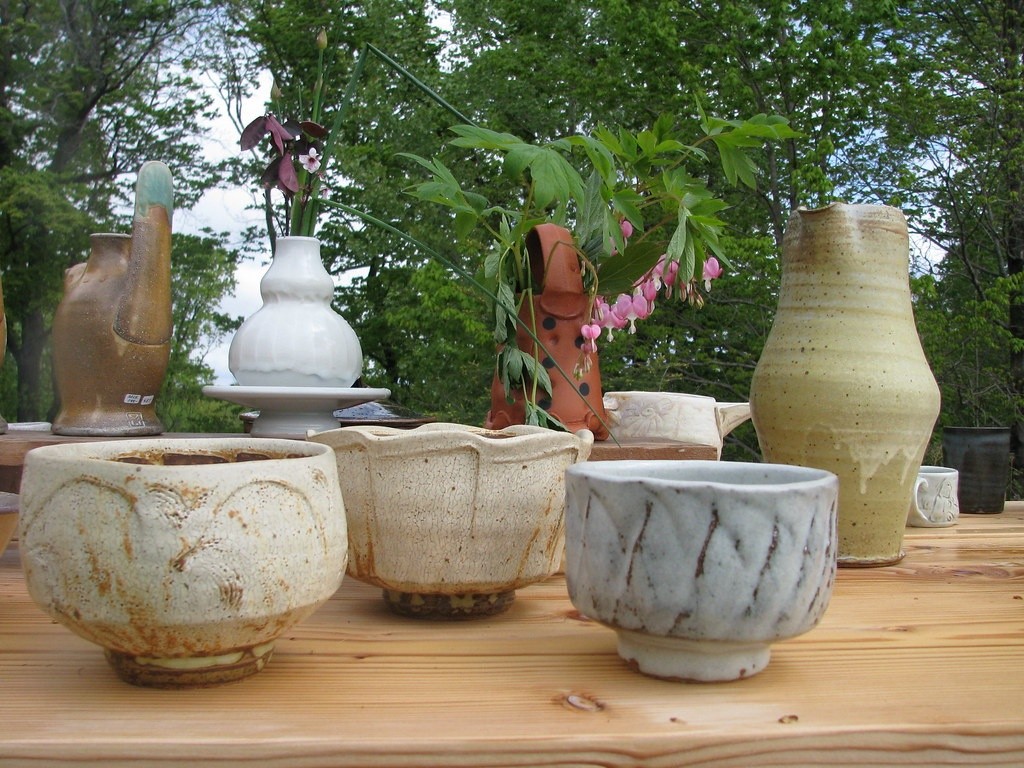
[0,513,1024,768]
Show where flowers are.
[239,21,624,448]
[384,89,805,434]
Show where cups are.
[906,465,960,527]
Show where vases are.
[16,435,352,697]
[944,424,1014,516]
[480,223,613,445]
[748,201,945,568]
[229,236,365,388]
[565,458,840,683]
[307,425,597,622]
